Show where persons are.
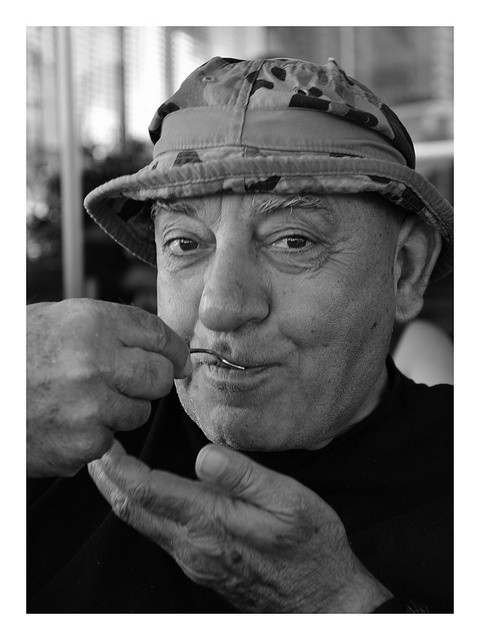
[28,57,454,614]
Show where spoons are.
[186,347,245,372]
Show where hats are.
[83,56,454,285]
